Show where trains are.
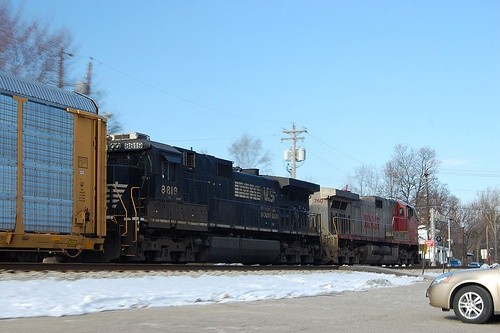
[0,71,421,268]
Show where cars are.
[467,261,481,268]
[446,259,464,268]
[425,266,500,323]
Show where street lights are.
[447,218,454,264]
[424,172,429,241]
